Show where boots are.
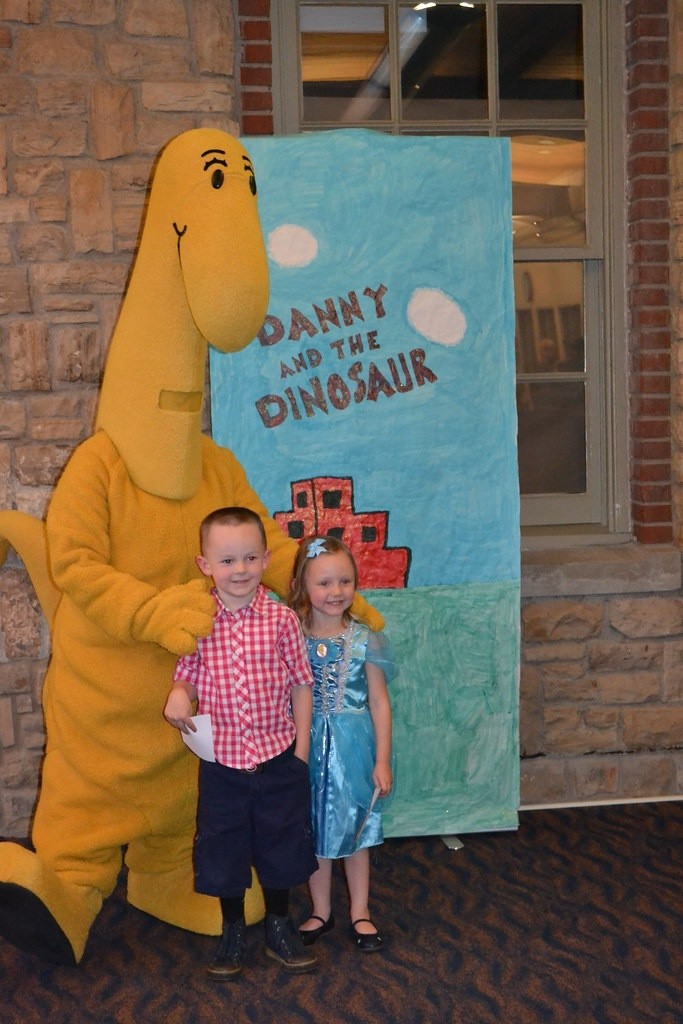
[264,913,317,973]
[207,916,248,980]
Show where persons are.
[284,534,403,953]
[162,505,321,981]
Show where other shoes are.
[349,919,383,952]
[297,910,335,945]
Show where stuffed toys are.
[0,122,387,968]
